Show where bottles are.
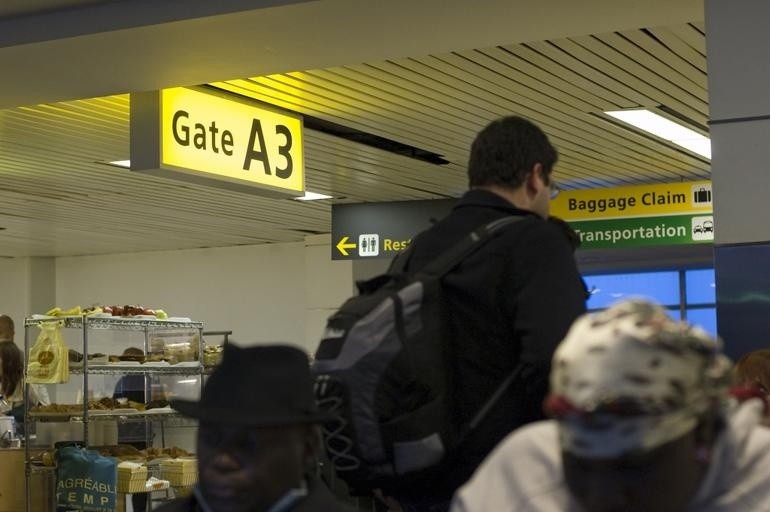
[150,383,168,401]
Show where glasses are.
[544,179,565,199]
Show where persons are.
[111,347,168,512]
[449,296,769,511]
[152,339,364,512]
[726,346,769,419]
[0,313,27,424]
[390,113,594,511]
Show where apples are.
[104,305,167,318]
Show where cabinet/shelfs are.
[24,314,206,512]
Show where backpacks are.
[314,210,549,497]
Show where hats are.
[554,300,733,461]
[169,341,339,427]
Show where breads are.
[98,444,191,462]
[42,451,53,466]
[30,397,121,412]
[68,349,105,362]
